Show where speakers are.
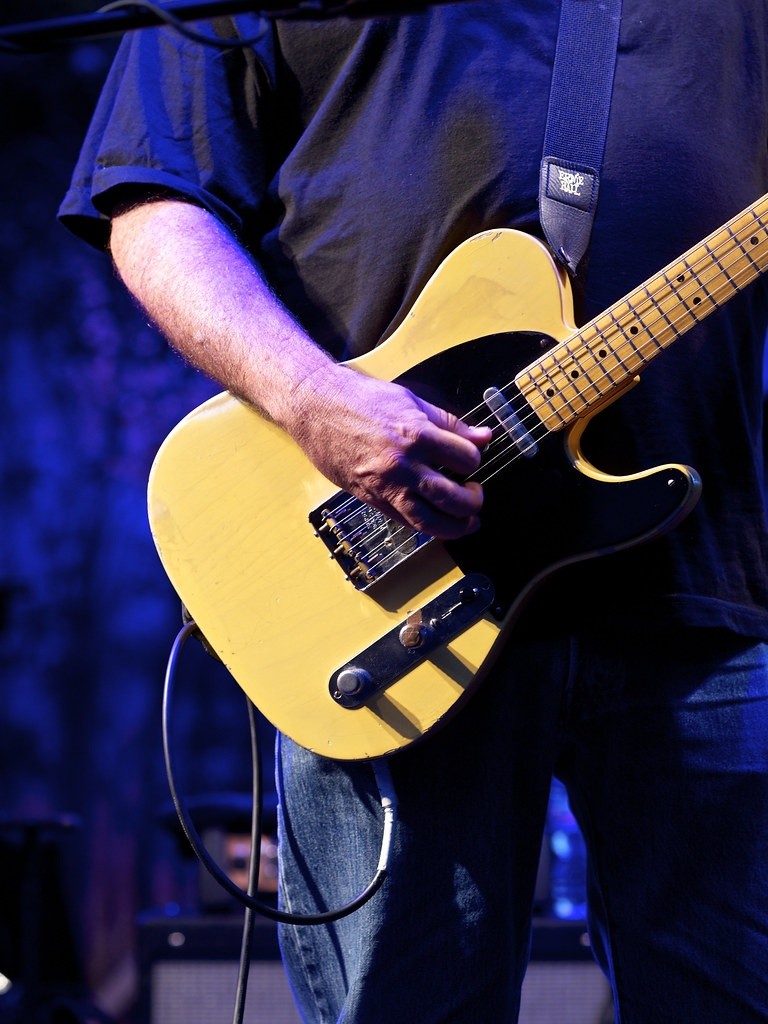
[135,914,614,1024]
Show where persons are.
[58,0,768,1024]
[93,826,276,1018]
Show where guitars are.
[141,191,768,765]
